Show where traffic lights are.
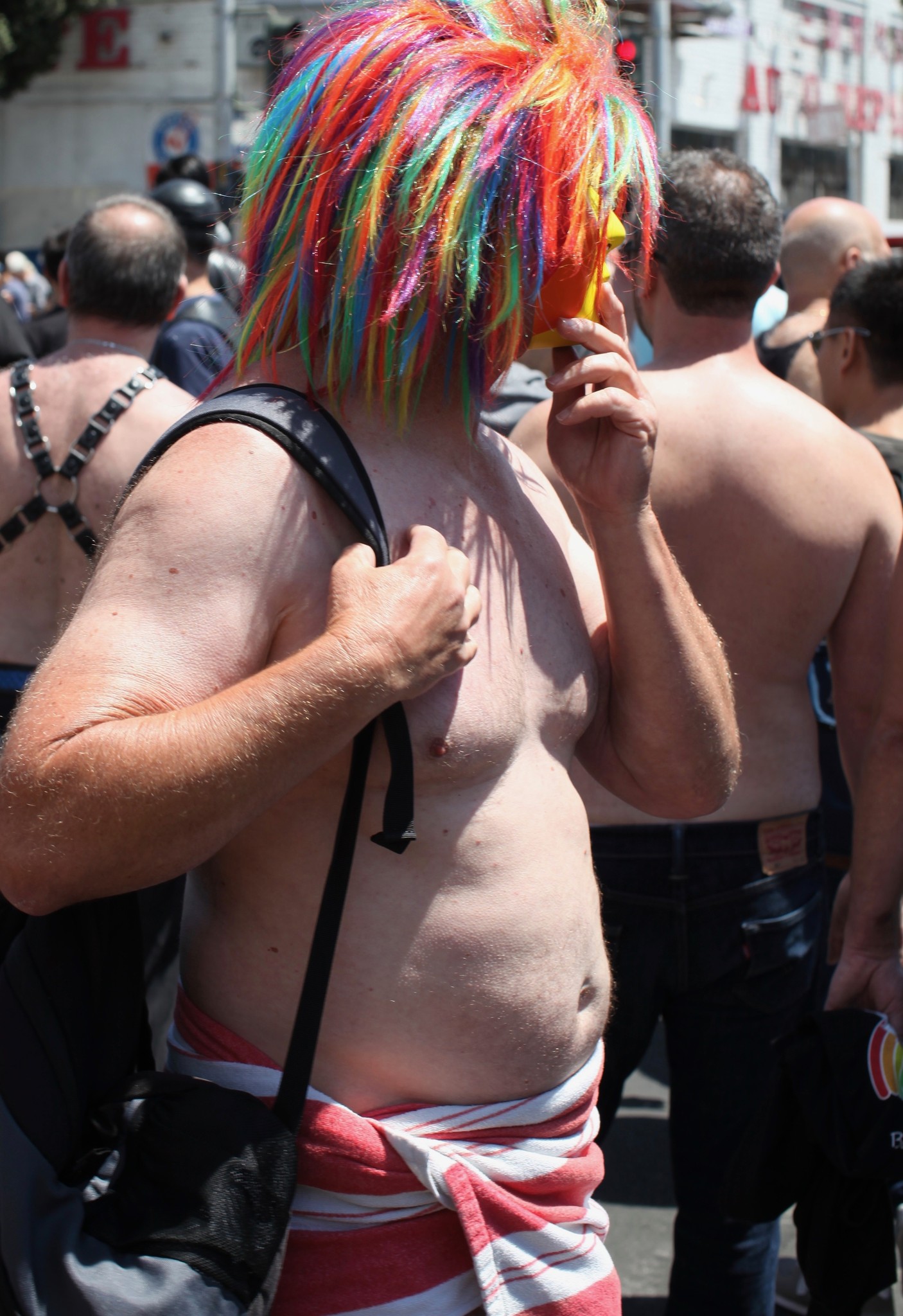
[266,6,303,68]
[615,37,639,66]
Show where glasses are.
[619,243,668,267]
[812,327,872,350]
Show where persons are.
[0,0,740,1316]
[0,148,903,1316]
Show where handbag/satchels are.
[0,1081,298,1315]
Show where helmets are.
[145,179,221,223]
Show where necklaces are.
[71,339,148,361]
